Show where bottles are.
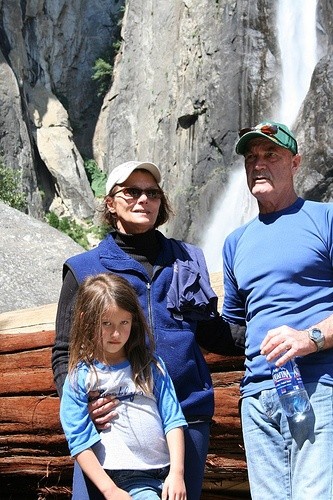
[267,349,311,417]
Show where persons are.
[192,121,333,500]
[59,273,188,500]
[51,161,214,500]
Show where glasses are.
[238,125,298,153]
[111,187,163,200]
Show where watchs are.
[306,326,326,352]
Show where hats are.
[235,121,298,157]
[106,161,161,196]
